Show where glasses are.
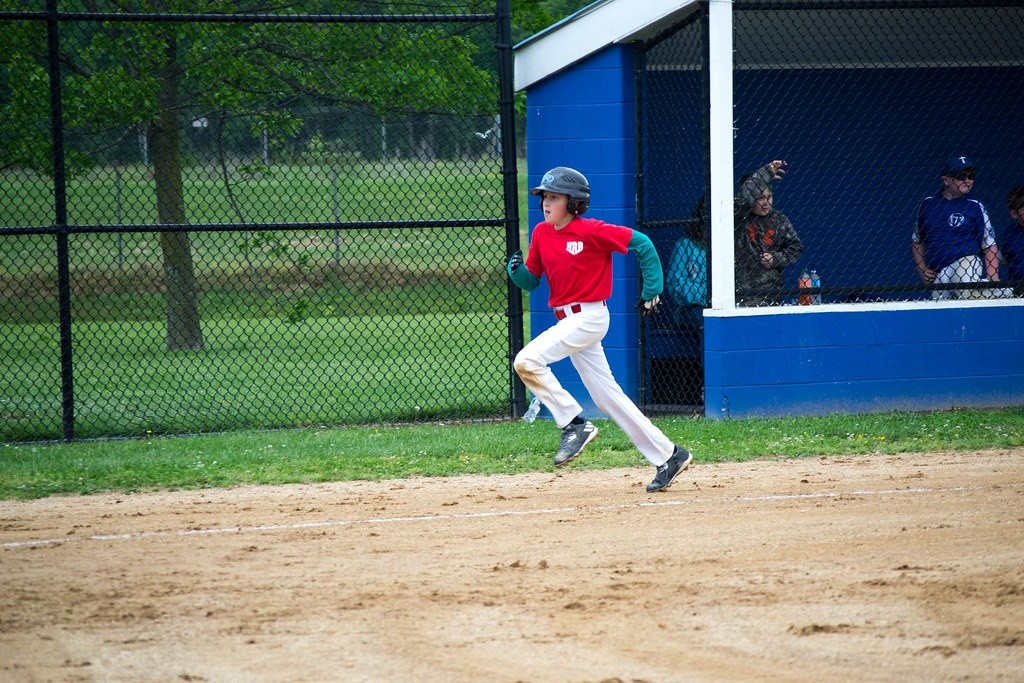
[945,172,975,181]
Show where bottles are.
[524,396,543,424]
[799,269,811,305]
[810,270,821,305]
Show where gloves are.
[633,295,664,319]
[511,248,523,271]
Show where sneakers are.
[554,418,598,467]
[646,445,692,493]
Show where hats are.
[936,149,974,175]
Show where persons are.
[734,160,804,306]
[507,166,693,493]
[664,204,707,402]
[911,153,1015,300]
[1001,186,1024,299]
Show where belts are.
[554,299,606,320]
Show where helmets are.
[530,167,591,215]
